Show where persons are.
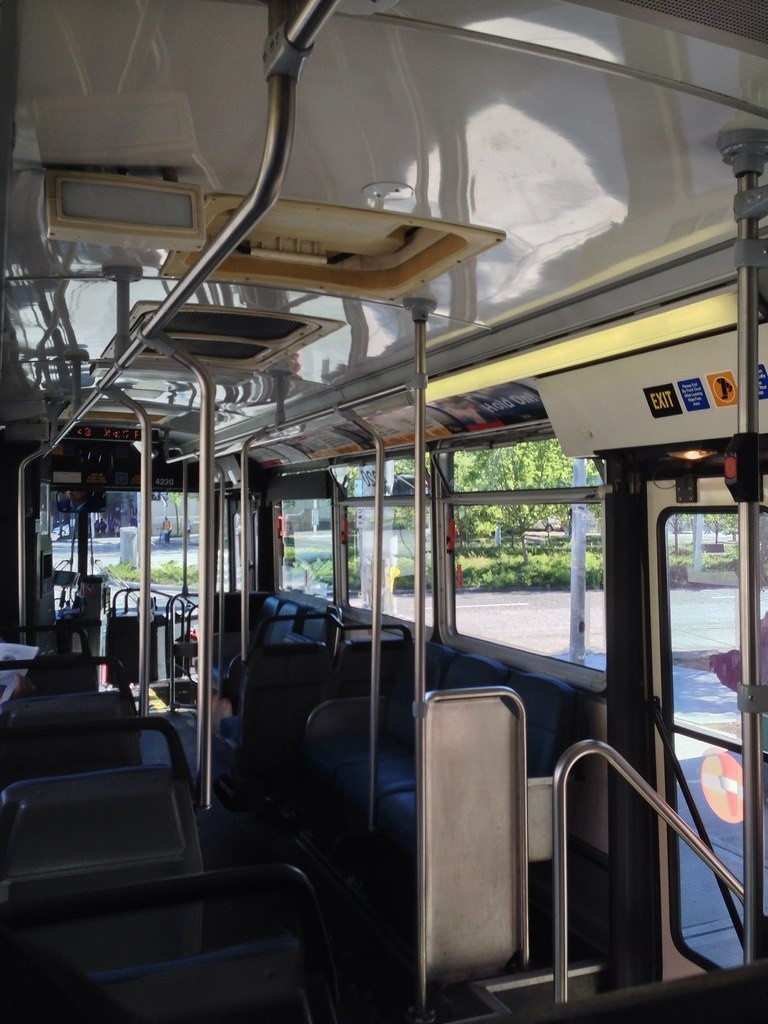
[59,489,99,510]
[13,651,98,698]
[94,515,190,544]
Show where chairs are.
[0,590,578,1024]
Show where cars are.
[53,524,70,537]
[517,514,564,532]
